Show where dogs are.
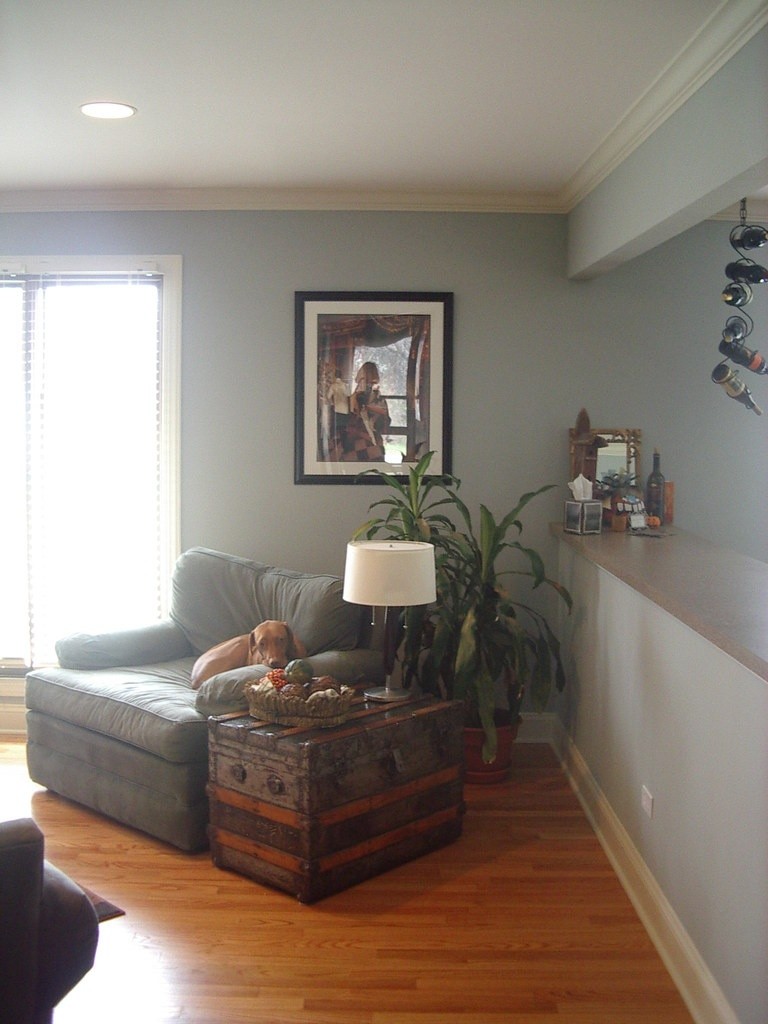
[190,620,305,690]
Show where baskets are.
[243,677,355,729]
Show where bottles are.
[725,319,745,343]
[646,453,665,526]
[732,227,768,248]
[721,288,752,307]
[712,365,762,415]
[719,339,768,375]
[726,262,768,283]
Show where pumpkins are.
[276,659,343,702]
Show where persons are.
[328,377,350,451]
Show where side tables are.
[206,681,466,905]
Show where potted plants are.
[348,451,573,784]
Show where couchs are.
[0,815,98,1024]
[24,547,394,852]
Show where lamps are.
[343,541,438,703]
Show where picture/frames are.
[293,291,453,487]
